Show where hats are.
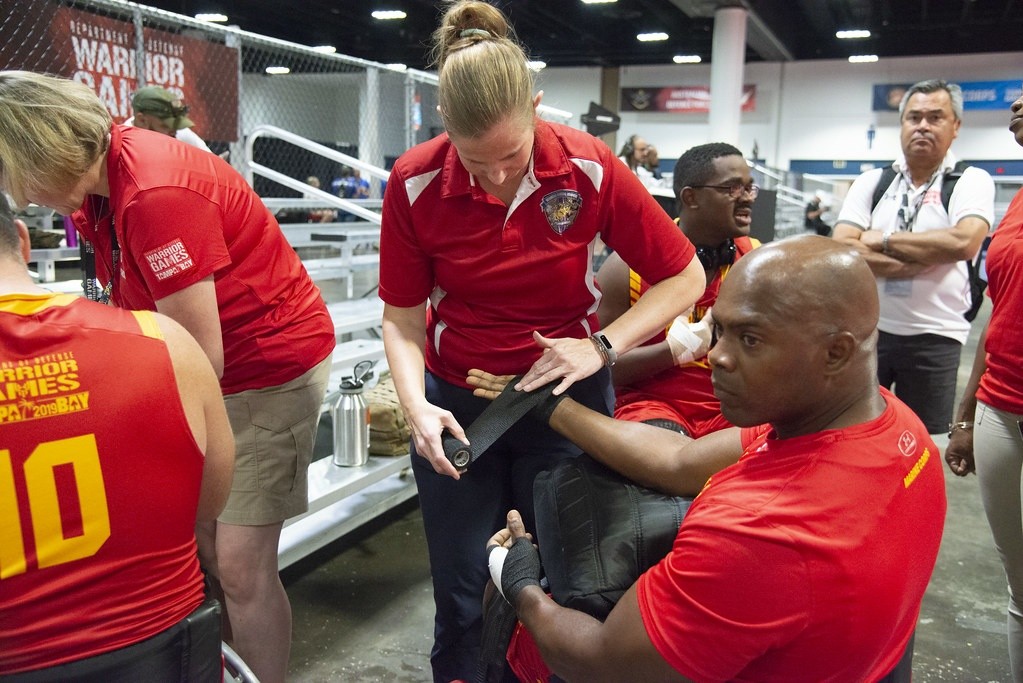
[130,87,195,131]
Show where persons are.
[123,88,229,162]
[0,70,337,683]
[615,135,672,198]
[378,1,707,683]
[805,190,831,237]
[944,95,1023,683]
[0,188,237,683]
[483,236,947,683]
[829,79,993,435]
[303,166,370,224]
[596,142,765,440]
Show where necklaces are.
[89,193,105,227]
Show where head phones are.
[697,238,737,271]
[625,135,636,156]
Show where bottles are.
[332,378,370,467]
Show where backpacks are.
[361,378,411,459]
[874,162,986,324]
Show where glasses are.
[687,182,760,200]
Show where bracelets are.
[589,336,611,370]
[947,421,975,438]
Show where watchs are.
[593,331,616,368]
[882,230,898,256]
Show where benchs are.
[6,197,418,571]
[773,189,839,244]
